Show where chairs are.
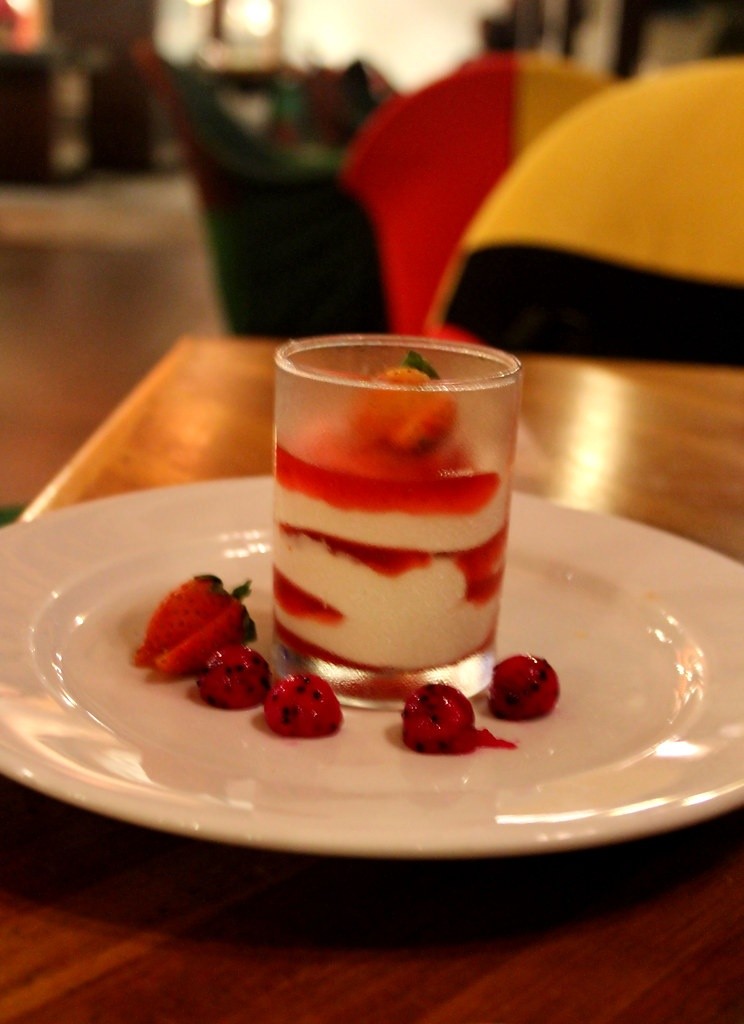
[179,57,743,377]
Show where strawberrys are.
[134,575,257,677]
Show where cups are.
[270,337,524,713]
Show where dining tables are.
[0,341,744,1024]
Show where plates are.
[0,473,744,851]
